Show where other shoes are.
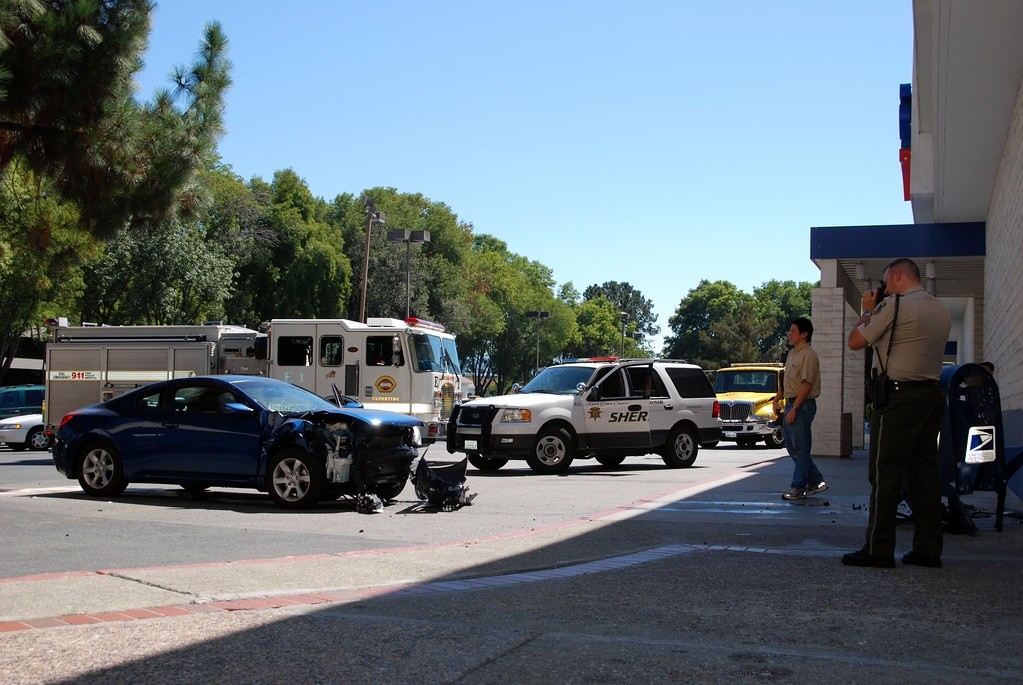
[947,503,980,536]
[901,550,942,568]
[957,478,973,494]
[841,549,896,568]
[940,502,951,534]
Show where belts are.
[902,380,940,389]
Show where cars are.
[0,383,46,420]
[0,414,54,451]
[51,372,427,510]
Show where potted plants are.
[864,403,873,444]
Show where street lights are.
[386,228,431,321]
[357,194,387,324]
[525,311,549,376]
[620,310,631,358]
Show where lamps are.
[926,279,937,297]
[854,261,865,280]
[925,260,936,278]
[863,278,872,292]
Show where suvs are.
[445,355,724,477]
[713,362,786,450]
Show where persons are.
[979,362,994,375]
[773,317,829,500]
[841,258,951,568]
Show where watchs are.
[863,311,871,315]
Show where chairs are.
[765,375,776,391]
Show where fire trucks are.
[38,316,464,448]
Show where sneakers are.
[805,479,828,495]
[782,481,808,499]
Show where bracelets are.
[791,405,798,410]
